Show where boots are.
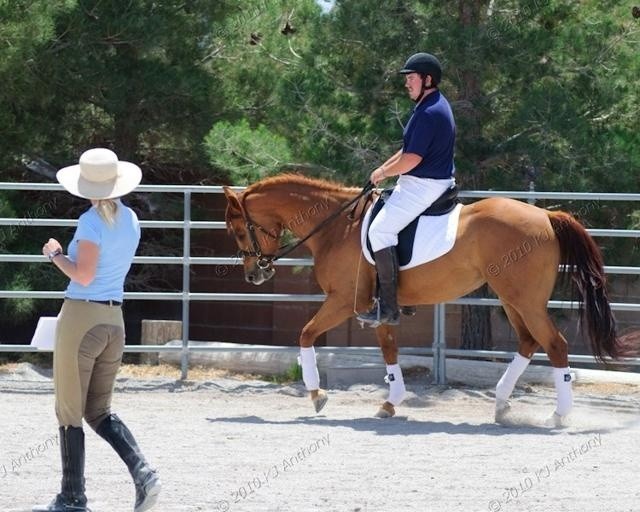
[358,247,416,323]
[96,414,162,512]
[32,425,87,512]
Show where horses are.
[222,173,632,427]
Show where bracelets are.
[381,167,388,179]
[48,249,62,262]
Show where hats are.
[56,148,143,200]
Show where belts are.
[89,300,121,305]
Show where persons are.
[31,147,165,512]
[355,52,456,326]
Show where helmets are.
[397,53,442,83]
